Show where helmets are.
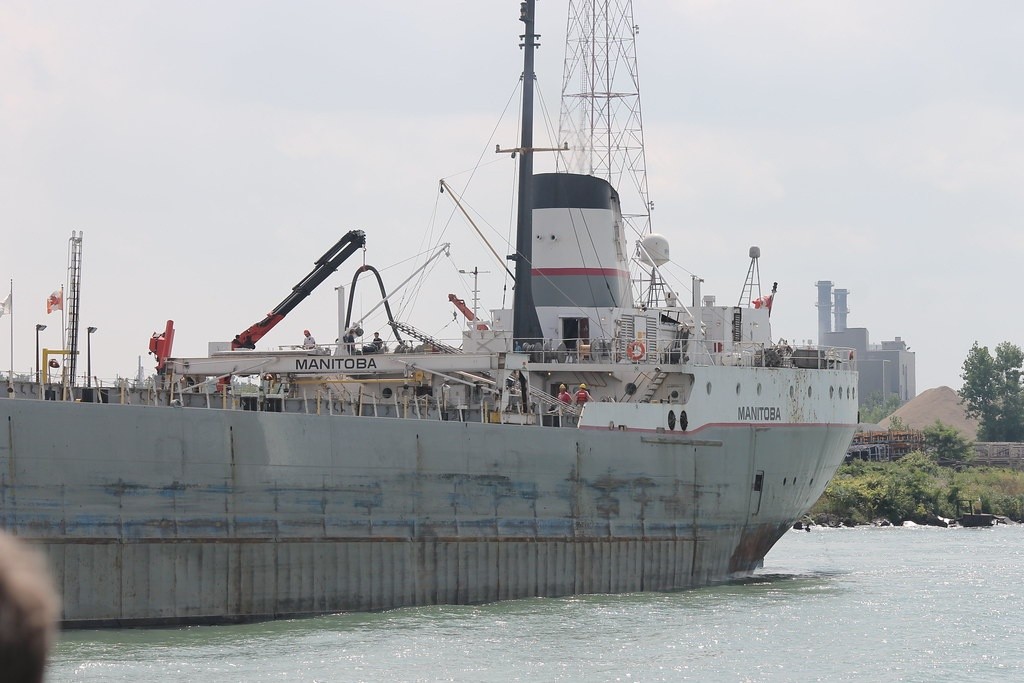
[560,384,566,389]
[580,383,587,389]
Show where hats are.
[304,330,309,335]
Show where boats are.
[0,0,860,622]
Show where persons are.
[544,339,555,363]
[347,329,357,355]
[231,334,255,351]
[572,383,594,406]
[302,330,315,350]
[0,529,58,683]
[558,384,572,405]
[371,332,383,350]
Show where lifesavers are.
[627,342,645,359]
